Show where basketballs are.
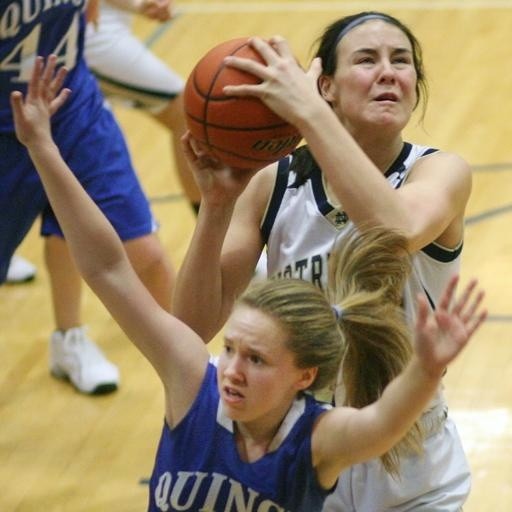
[184,37,304,170]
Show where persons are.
[10,52,487,512]
[0,0,176,315]
[0,204,121,396]
[81,0,203,216]
[170,10,475,512]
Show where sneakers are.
[48,322,121,397]
[6,256,35,286]
